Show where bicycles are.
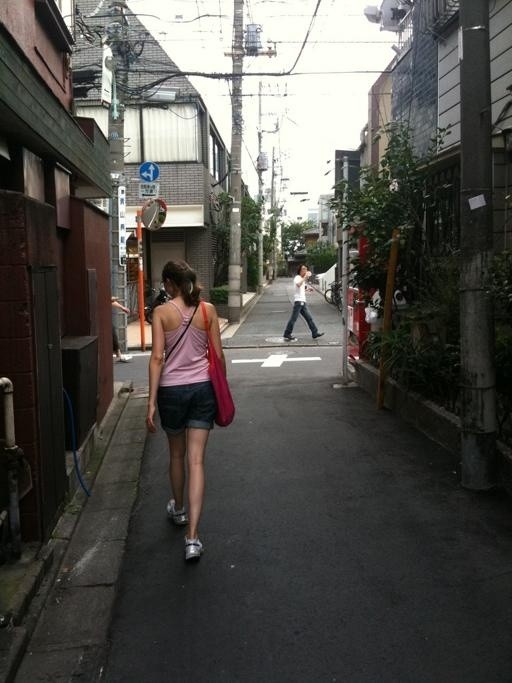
[325,281,342,312]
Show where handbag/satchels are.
[206,349,236,427]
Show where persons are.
[282,264,326,340]
[145,259,226,562]
[111,295,131,363]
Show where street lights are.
[105,56,126,354]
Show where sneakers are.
[312,331,324,339]
[283,332,295,339]
[183,535,203,562]
[114,353,132,362]
[166,497,188,525]
